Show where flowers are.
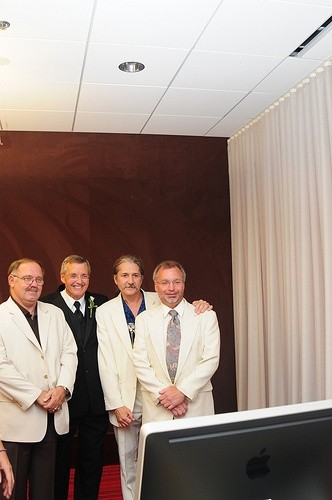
[87,296,97,319]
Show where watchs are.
[61,386,69,394]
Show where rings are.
[53,408,58,411]
[207,303,210,305]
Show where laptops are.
[135,399,332,500]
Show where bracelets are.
[0,449,7,452]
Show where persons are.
[38,255,110,500]
[0,258,78,500]
[0,441,15,499]
[95,255,213,500]
[133,261,220,423]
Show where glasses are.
[156,280,183,287]
[11,274,44,285]
[65,272,90,279]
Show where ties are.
[73,301,83,325]
[166,310,181,384]
